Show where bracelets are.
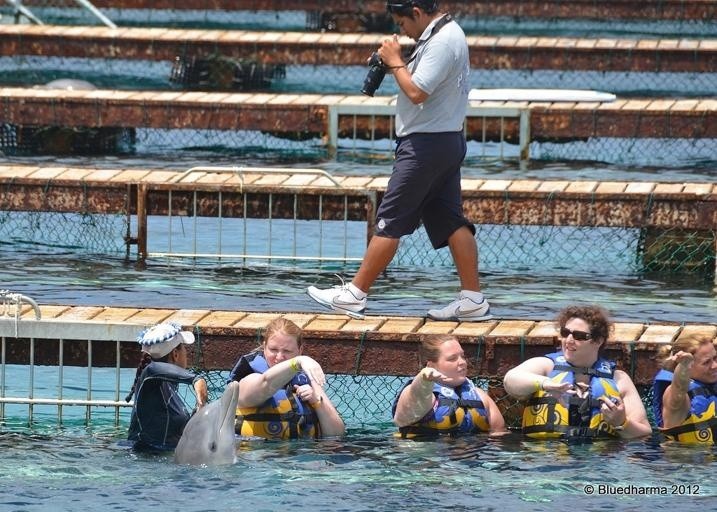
[534,374,548,390]
[290,357,299,373]
[309,395,323,409]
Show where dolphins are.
[172,380,240,466]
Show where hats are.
[137,320,196,359]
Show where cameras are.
[360,52,390,97]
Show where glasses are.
[560,325,594,341]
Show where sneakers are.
[305,282,367,320]
[426,291,492,322]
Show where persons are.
[127,324,208,458]
[653,325,717,446]
[503,306,652,442]
[226,317,345,444]
[391,334,509,437]
[306,0,494,323]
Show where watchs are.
[613,417,629,432]
